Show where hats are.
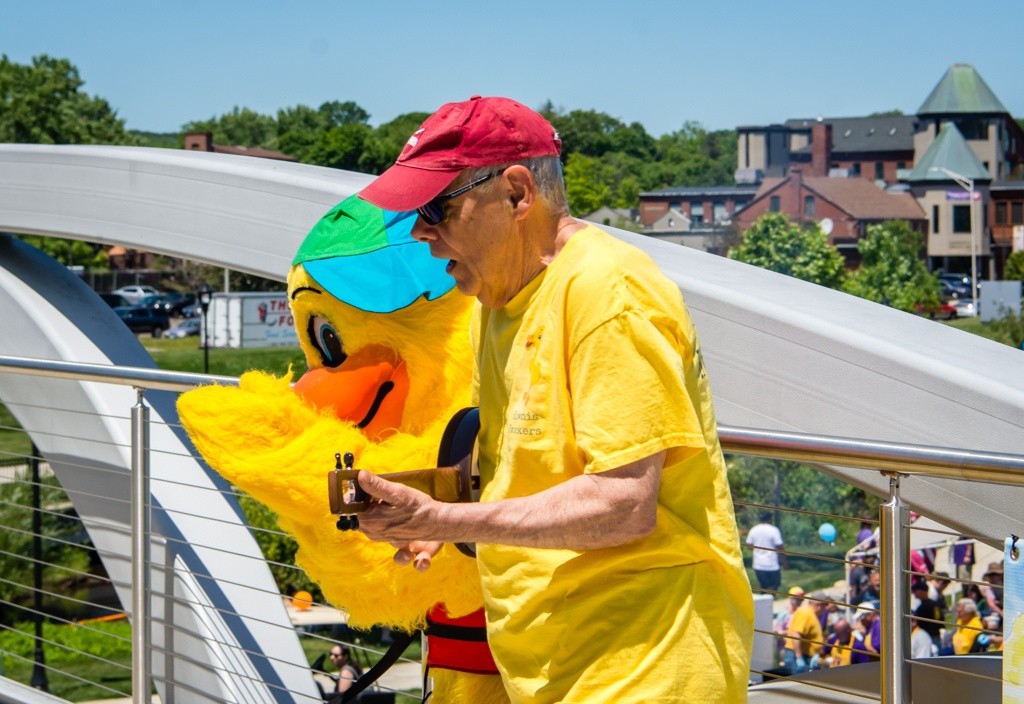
[360,94,563,212]
[854,601,875,621]
[789,587,804,598]
[760,511,774,520]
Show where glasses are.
[415,168,506,226]
[329,652,342,657]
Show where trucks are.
[202,291,300,346]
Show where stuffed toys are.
[175,186,511,704]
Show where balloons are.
[818,521,837,546]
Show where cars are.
[934,274,980,298]
[903,298,981,321]
[100,285,200,340]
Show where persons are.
[745,510,785,597]
[358,94,755,704]
[772,511,1004,675]
[329,642,363,695]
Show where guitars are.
[324,404,481,560]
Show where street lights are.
[199,285,212,377]
[929,164,980,318]
